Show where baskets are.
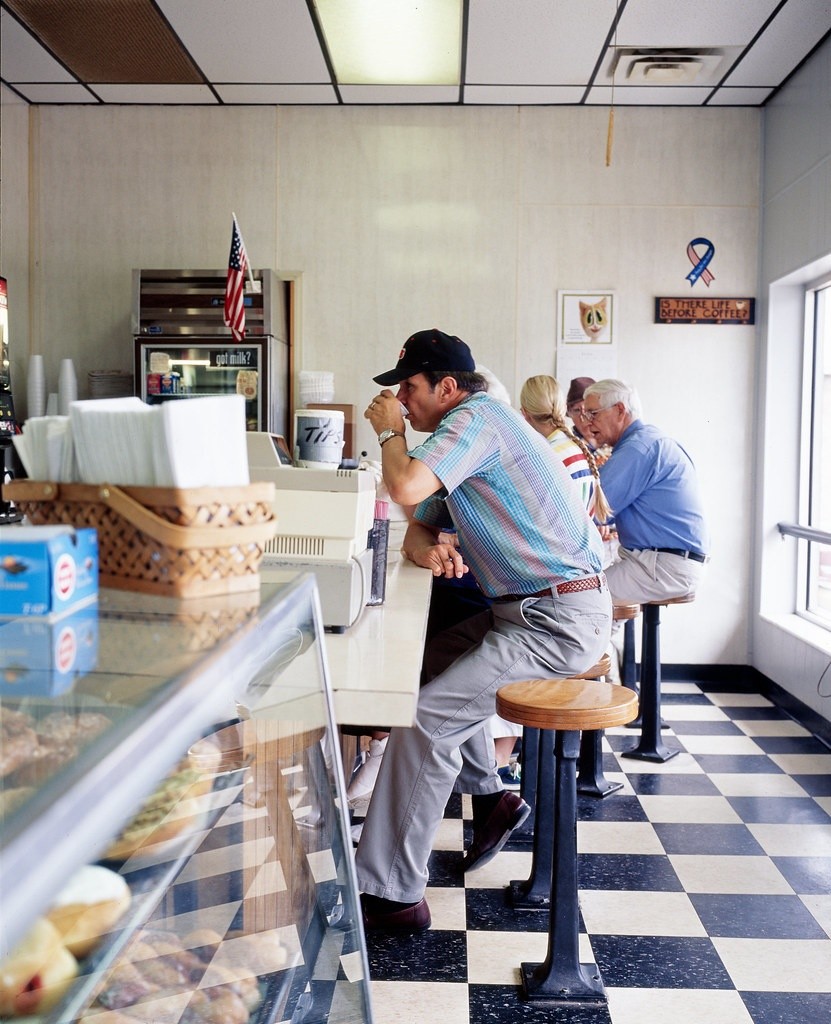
[2,478,276,599]
[70,606,260,707]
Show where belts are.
[490,575,606,605]
[626,546,704,563]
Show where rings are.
[370,402,377,408]
[446,557,452,562]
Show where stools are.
[496,584,696,1003]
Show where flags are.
[222,221,248,343]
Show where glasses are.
[579,404,617,422]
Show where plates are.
[88,369,133,399]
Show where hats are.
[372,329,475,387]
[566,377,596,405]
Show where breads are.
[0,707,288,1024]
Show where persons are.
[348,569,488,809]
[581,379,711,639]
[563,376,612,689]
[521,376,621,687]
[320,329,613,932]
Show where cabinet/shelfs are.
[0,570,371,1024]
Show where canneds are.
[148,371,180,394]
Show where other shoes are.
[346,735,392,810]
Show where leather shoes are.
[458,792,531,873]
[362,897,429,931]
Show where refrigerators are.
[130,267,288,433]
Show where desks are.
[0,463,435,730]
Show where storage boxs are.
[0,595,99,698]
[0,528,100,623]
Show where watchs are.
[378,428,406,447]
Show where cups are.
[400,402,409,416]
[27,354,78,416]
